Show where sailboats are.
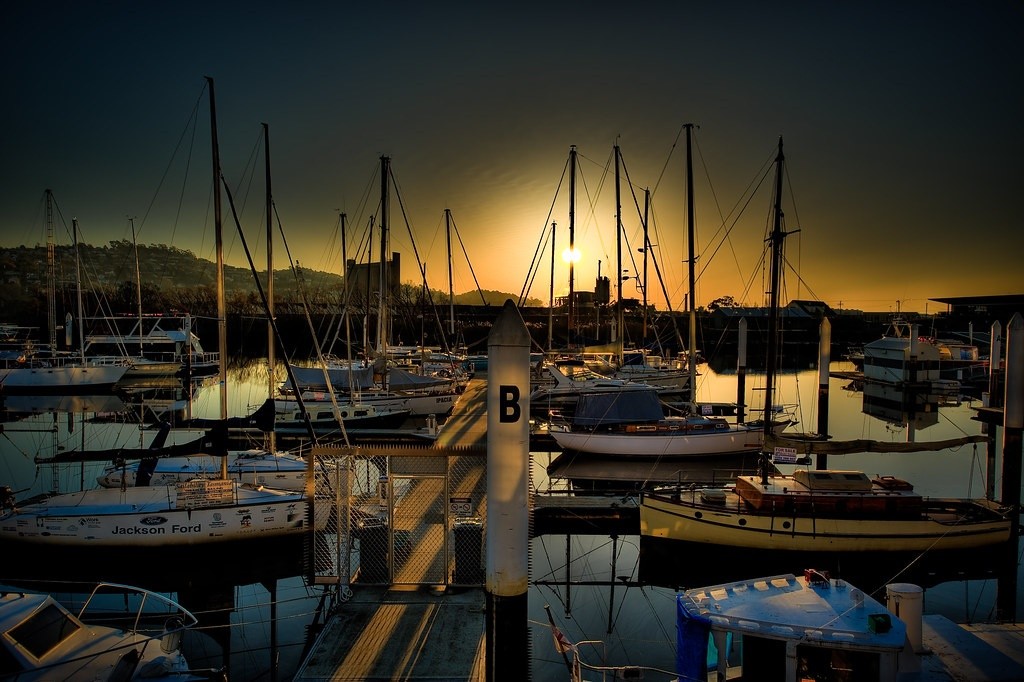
[550,123,793,455]
[636,135,1017,552]
[102,115,384,503]
[34,577,279,681]
[4,60,337,543]
[0,75,741,423]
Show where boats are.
[0,577,227,682]
[937,342,985,373]
